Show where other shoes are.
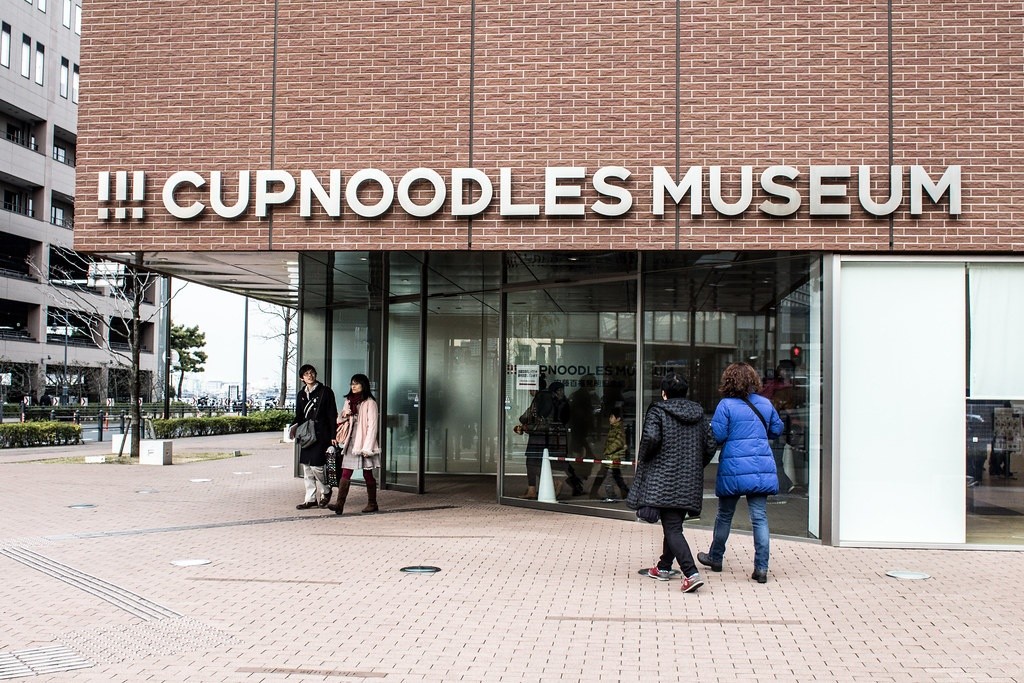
[788,485,795,494]
[766,495,788,504]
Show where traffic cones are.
[537,449,558,504]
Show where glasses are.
[304,371,315,376]
[350,383,359,386]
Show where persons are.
[754,359,809,504]
[518,374,630,500]
[697,361,784,583]
[288,364,338,509]
[327,374,386,515]
[966,400,1016,488]
[39,391,52,406]
[626,372,718,593]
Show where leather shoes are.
[697,552,722,572]
[752,571,767,583]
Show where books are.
[289,423,298,440]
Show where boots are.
[362,479,378,512]
[554,479,564,498]
[328,479,350,514]
[517,486,538,500]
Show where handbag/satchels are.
[519,389,556,434]
[295,419,316,448]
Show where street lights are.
[51,311,69,405]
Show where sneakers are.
[319,484,333,508]
[648,567,670,581]
[681,574,704,593]
[296,499,319,509]
